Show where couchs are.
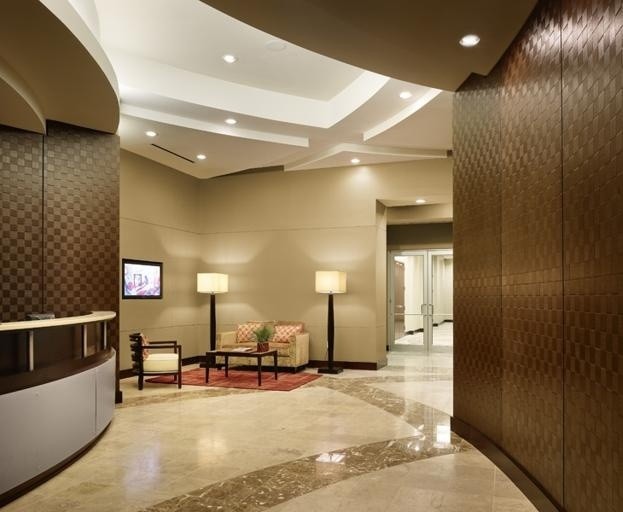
[215,330,310,374]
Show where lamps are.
[313,270,352,374]
[197,272,230,368]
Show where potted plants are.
[254,328,272,352]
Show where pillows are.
[237,323,264,346]
[273,324,303,342]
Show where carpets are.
[144,367,322,392]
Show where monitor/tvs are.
[25,314,55,321]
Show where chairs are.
[129,332,183,391]
